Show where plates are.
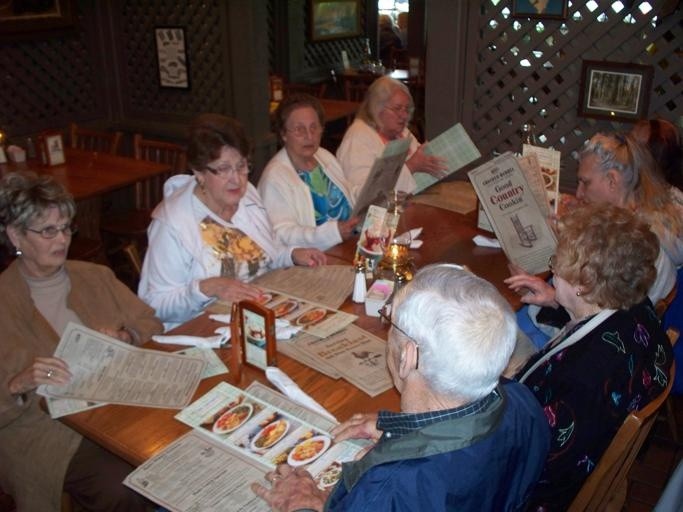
[250,421,290,453]
[295,306,327,327]
[272,300,298,318]
[211,402,253,435]
[286,435,333,469]
[363,278,395,318]
[542,171,554,188]
[259,294,271,306]
[318,467,344,490]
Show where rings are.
[47,370,53,378]
[270,477,280,483]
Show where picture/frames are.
[511,0,568,21]
[573,58,655,123]
[153,25,193,94]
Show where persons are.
[0,169,164,512]
[335,76,449,201]
[257,94,365,253]
[252,264,551,512]
[138,113,328,335]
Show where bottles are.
[357,37,371,71]
[372,59,385,76]
[394,275,405,293]
[23,137,37,158]
[352,261,366,304]
[376,190,411,270]
[519,123,536,152]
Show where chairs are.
[651,270,682,317]
[267,1,431,147]
[0,124,200,261]
[122,227,154,278]
[565,329,679,510]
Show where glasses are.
[386,103,415,117]
[546,255,557,272]
[284,124,326,136]
[22,224,78,239]
[204,161,254,176]
[375,305,420,370]
